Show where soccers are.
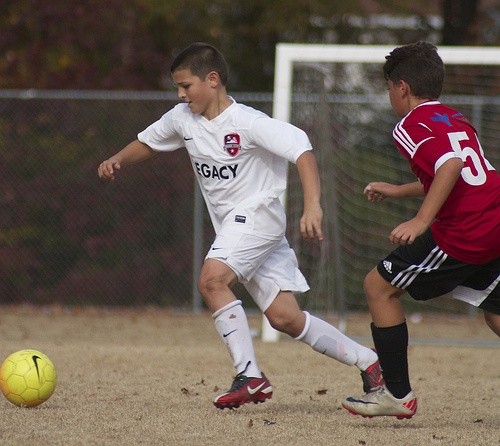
[0,349,57,408]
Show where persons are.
[96,44,384,410]
[341,42,500,421]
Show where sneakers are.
[361,347,385,395]
[213,371,273,408]
[341,386,416,419]
